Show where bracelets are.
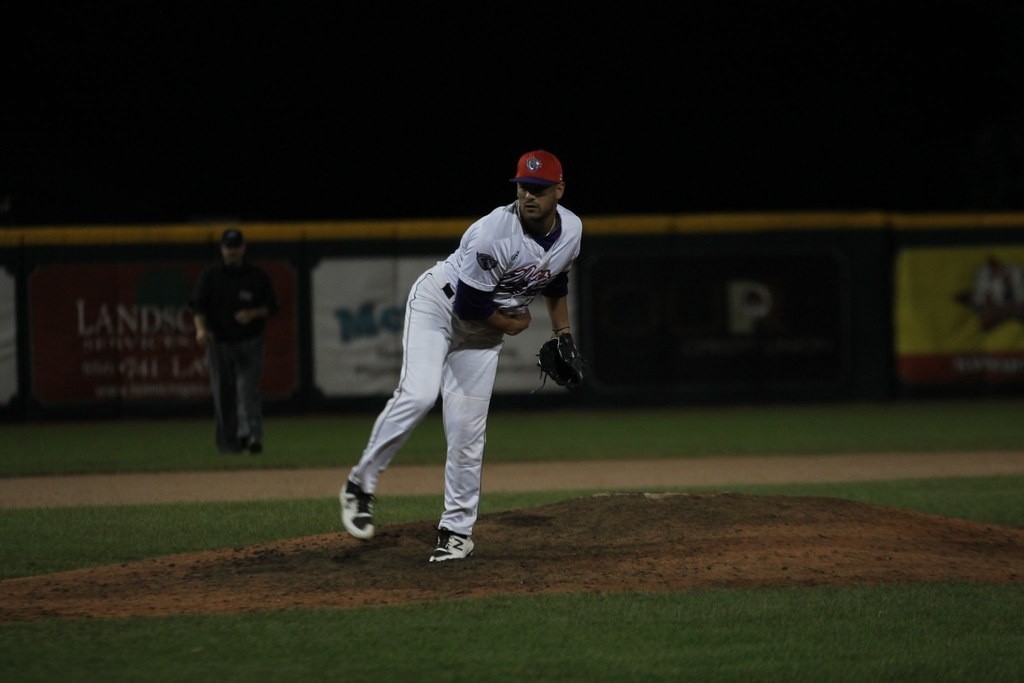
[552,326,570,335]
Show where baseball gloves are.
[537,336,589,389]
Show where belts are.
[443,283,456,299]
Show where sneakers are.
[429,526,475,562]
[339,480,375,539]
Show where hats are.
[509,150,562,186]
[222,230,243,248]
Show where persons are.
[339,150,583,562]
[191,229,279,454]
